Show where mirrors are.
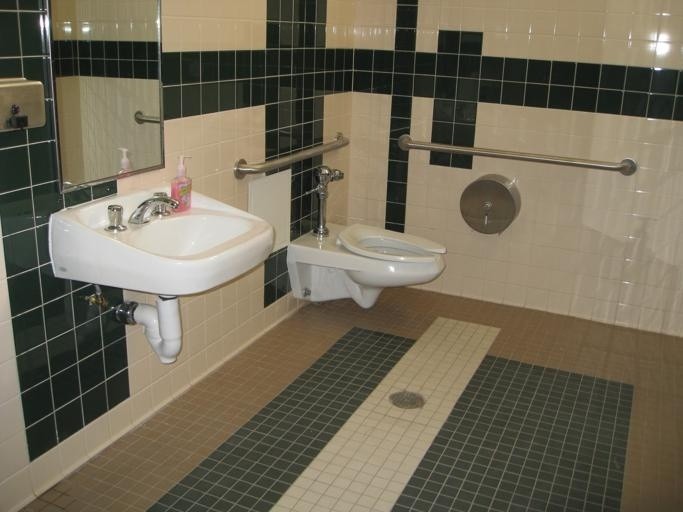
[38,1,170,194]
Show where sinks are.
[47,182,274,297]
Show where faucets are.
[129,194,180,224]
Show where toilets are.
[286,223,446,310]
